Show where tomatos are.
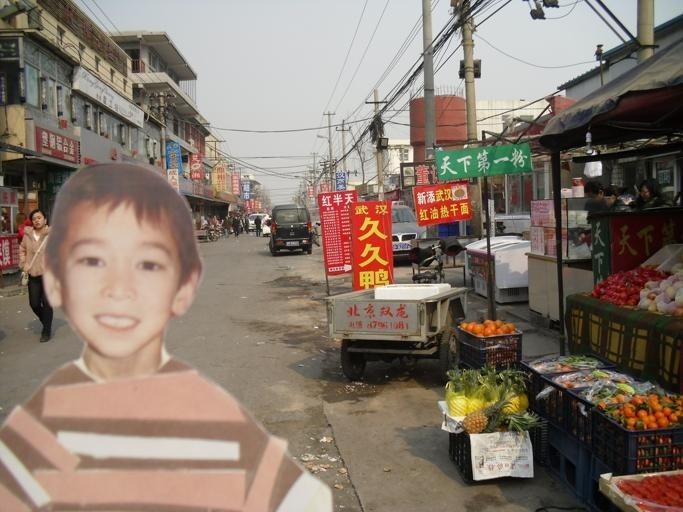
[590,267,664,305]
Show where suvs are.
[390,204,429,263]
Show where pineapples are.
[444,383,530,434]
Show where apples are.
[638,276,683,316]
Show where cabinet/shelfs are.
[560,288,682,394]
[524,194,594,322]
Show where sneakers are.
[39,332,50,343]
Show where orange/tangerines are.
[530,362,682,470]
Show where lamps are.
[583,125,595,155]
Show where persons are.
[18,208,53,343]
[16,214,33,244]
[576,176,674,248]
[0,162,335,512]
[207,210,272,240]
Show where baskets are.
[447,425,475,488]
[516,349,683,512]
[457,323,524,374]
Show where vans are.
[261,214,271,237]
[266,203,311,256]
[246,213,268,232]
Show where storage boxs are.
[372,282,451,303]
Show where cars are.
[560,208,591,244]
[478,211,531,242]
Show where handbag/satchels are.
[19,271,30,287]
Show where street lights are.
[316,131,334,193]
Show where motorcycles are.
[326,240,469,381]
[205,223,219,243]
